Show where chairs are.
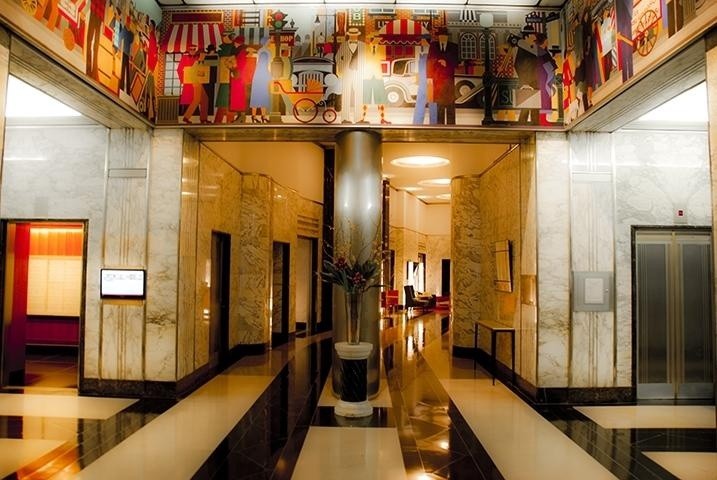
[404,285,430,315]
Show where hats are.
[234,35,245,44]
[549,46,562,53]
[260,37,269,45]
[346,27,361,36]
[435,27,452,36]
[189,43,198,49]
[520,25,536,34]
[206,45,216,50]
[370,34,383,39]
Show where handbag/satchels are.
[574,67,586,81]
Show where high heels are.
[231,116,245,125]
[252,115,260,123]
[262,116,270,123]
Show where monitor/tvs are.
[100,268,146,300]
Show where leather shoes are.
[342,120,352,124]
[355,121,370,124]
[183,117,192,123]
[200,120,211,124]
[381,119,391,125]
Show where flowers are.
[314,207,395,339]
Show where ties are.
[373,46,375,54]
[442,43,444,52]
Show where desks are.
[474,319,516,386]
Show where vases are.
[341,289,367,345]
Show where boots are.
[539,113,552,126]
